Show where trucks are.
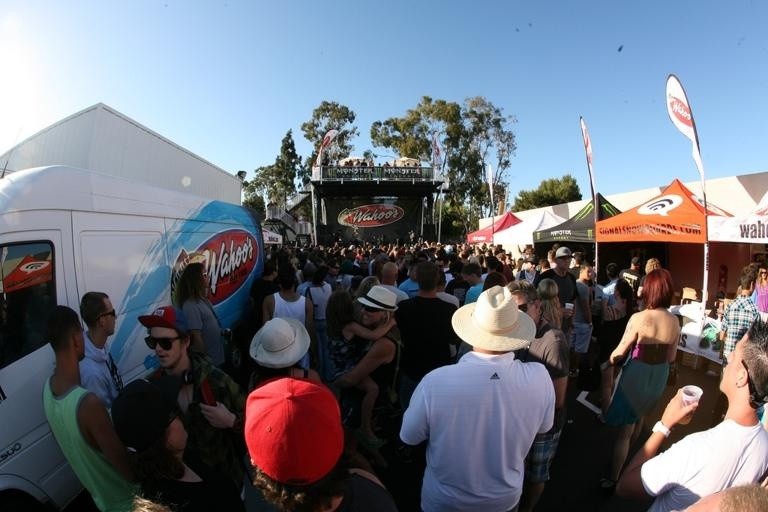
[0,102,268,511]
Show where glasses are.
[145,334,180,351]
[96,309,116,320]
[740,359,768,408]
[360,304,383,313]
[517,299,533,311]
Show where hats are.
[556,247,574,259]
[451,286,536,351]
[112,375,182,445]
[246,376,344,484]
[357,286,398,311]
[138,307,190,336]
[250,318,310,368]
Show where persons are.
[43,228,767,511]
[332,157,424,179]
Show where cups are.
[676,384,702,426]
[565,302,574,310]
